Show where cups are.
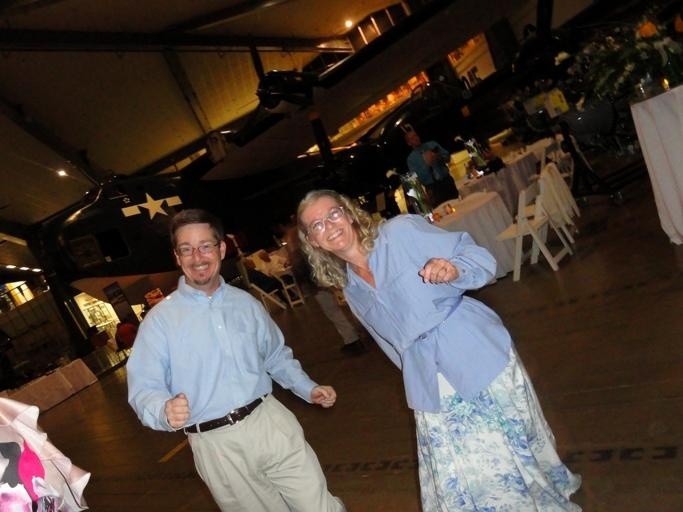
[442,204,453,216]
[636,82,650,99]
[432,213,441,222]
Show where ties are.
[420,149,441,182]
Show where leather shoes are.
[340,339,369,356]
[365,333,376,346]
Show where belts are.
[311,288,322,296]
[425,174,450,188]
[183,393,269,433]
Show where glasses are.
[306,206,345,233]
[176,242,219,256]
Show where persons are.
[472,145,505,177]
[244,258,300,306]
[124,208,346,512]
[295,188,583,512]
[115,322,138,350]
[403,130,459,207]
[256,250,289,275]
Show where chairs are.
[513,140,582,245]
[271,268,306,309]
[528,140,564,183]
[249,281,289,311]
[495,182,574,282]
